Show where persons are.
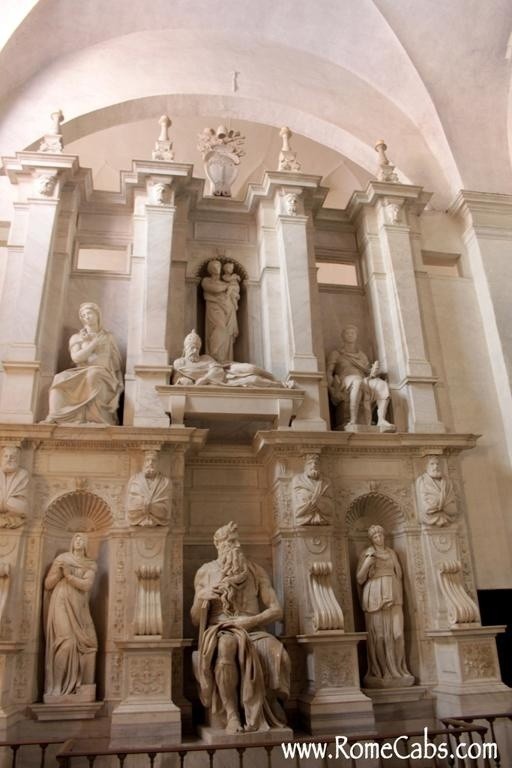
[170,331,298,389]
[189,521,292,736]
[416,454,460,526]
[37,302,126,424]
[356,524,416,689]
[200,257,243,367]
[1,445,30,528]
[290,459,336,526]
[124,448,175,527]
[327,324,398,433]
[43,531,99,705]
